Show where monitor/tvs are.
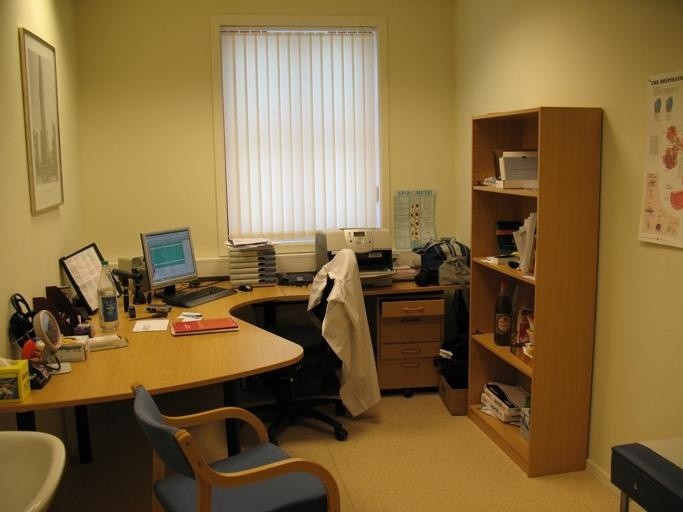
[140,226,199,298]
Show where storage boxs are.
[437,375,467,416]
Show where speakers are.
[118,255,149,291]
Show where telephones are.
[276,272,314,287]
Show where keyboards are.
[167,285,237,308]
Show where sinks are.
[0,430,67,511]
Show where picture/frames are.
[17,26,66,218]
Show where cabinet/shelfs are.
[375,297,445,398]
[466,106,604,479]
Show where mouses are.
[239,285,253,292]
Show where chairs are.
[128,383,342,512]
[237,274,349,447]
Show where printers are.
[315,227,392,287]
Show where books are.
[223,237,278,289]
[169,316,240,335]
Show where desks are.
[0,280,465,466]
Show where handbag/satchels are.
[412,237,470,286]
[434,334,468,389]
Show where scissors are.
[127,312,168,321]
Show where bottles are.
[493,278,513,346]
[97,260,120,332]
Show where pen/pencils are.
[183,315,202,317]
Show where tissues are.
[1,355,32,405]
